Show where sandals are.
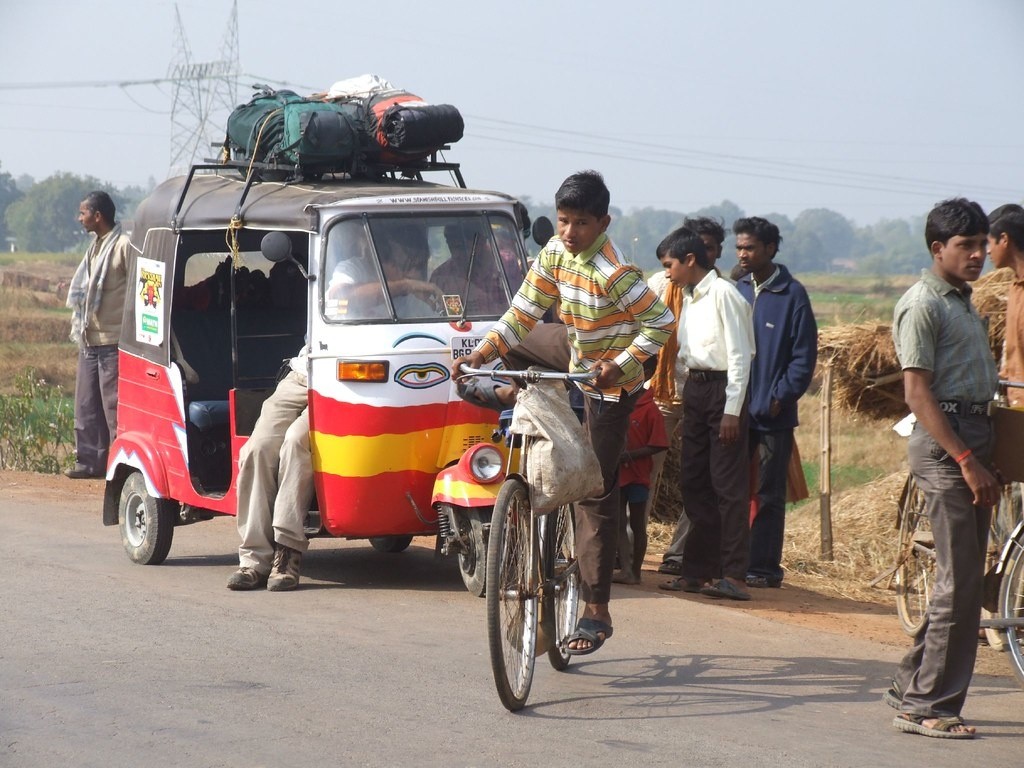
[881,688,901,711]
[893,714,975,738]
[702,580,752,599]
[659,576,701,592]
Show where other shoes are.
[64,468,105,479]
[658,559,685,574]
[613,570,641,584]
[745,574,781,588]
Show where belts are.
[939,400,988,416]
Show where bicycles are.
[866,380,1024,680]
[454,362,604,710]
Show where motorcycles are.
[101,159,554,597]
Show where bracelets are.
[955,449,972,464]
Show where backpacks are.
[226,73,465,183]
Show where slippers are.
[566,618,614,655]
[456,382,507,412]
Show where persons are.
[881,196,1024,740]
[450,170,678,652]
[63,191,131,478]
[429,211,819,601]
[228,233,447,593]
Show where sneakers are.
[226,568,266,590]
[266,542,302,591]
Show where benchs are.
[173,251,308,432]
